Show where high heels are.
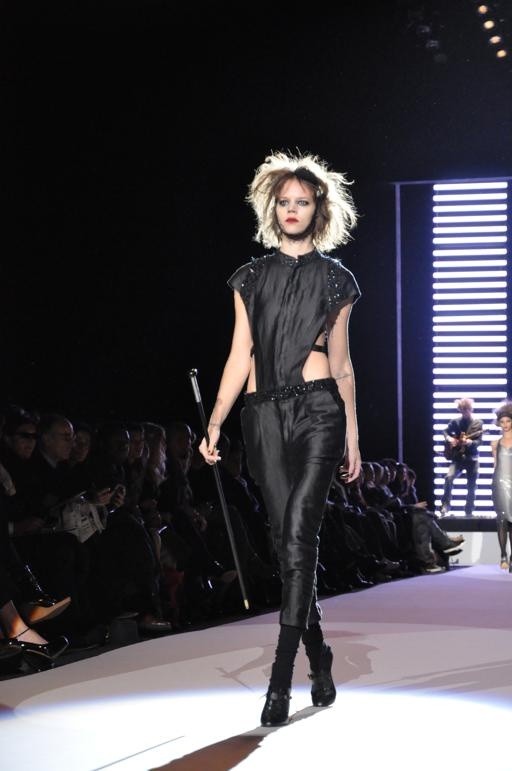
[8,633,69,671]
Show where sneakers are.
[441,506,448,513]
[466,510,473,517]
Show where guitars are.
[444,429,484,460]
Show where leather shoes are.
[440,535,464,551]
[500,555,509,569]
[352,575,376,587]
[140,614,172,632]
[375,558,400,571]
[425,563,446,573]
[261,662,294,726]
[305,641,336,706]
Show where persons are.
[489,403,511,569]
[0,403,462,678]
[441,398,482,515]
[195,150,365,726]
